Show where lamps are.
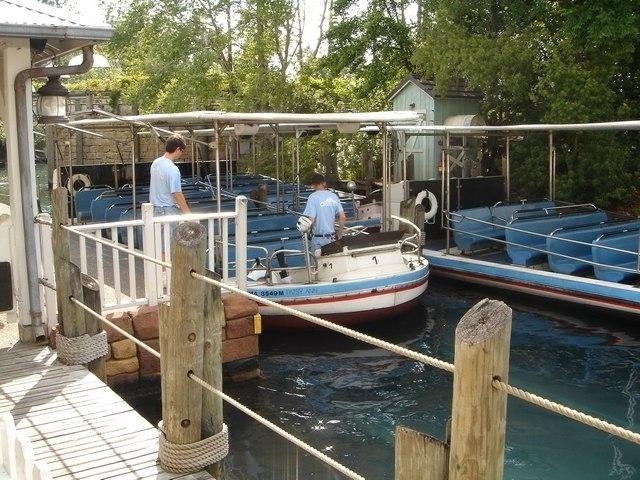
[37,76,71,124]
[338,122,360,134]
[234,123,259,140]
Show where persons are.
[295,174,346,265]
[148,133,191,290]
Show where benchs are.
[451,201,640,282]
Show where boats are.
[45,111,430,328]
[359,122,640,315]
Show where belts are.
[311,232,337,237]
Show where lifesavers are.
[68,174,91,195]
[415,190,438,220]
